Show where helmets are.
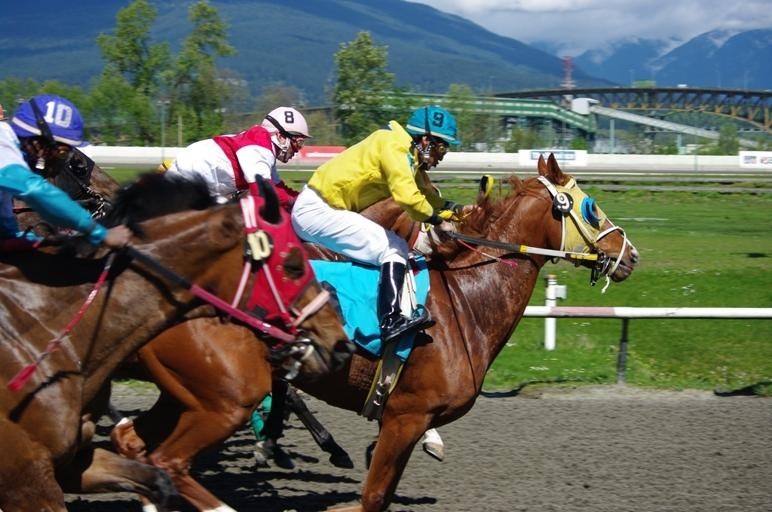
[10,94,90,148]
[405,104,462,145]
[261,106,313,139]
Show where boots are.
[377,262,436,345]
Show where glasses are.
[291,138,305,152]
[437,144,448,160]
[53,146,76,166]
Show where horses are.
[0,136,641,510]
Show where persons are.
[291,105,482,345]
[165,107,312,207]
[0,95,133,250]
